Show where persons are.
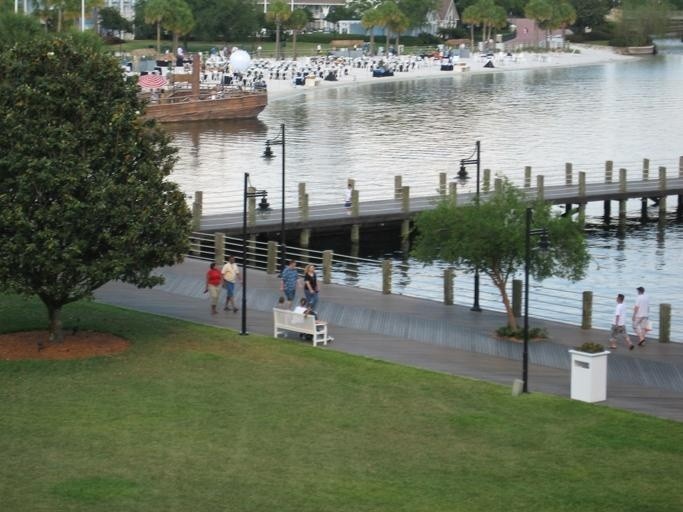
[276,260,321,340]
[203,254,243,315]
[344,184,353,214]
[607,287,652,352]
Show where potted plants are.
[569,340,612,403]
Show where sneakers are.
[223,306,239,314]
[637,339,645,346]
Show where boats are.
[135,53,268,123]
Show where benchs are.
[271,306,330,347]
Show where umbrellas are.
[137,74,168,98]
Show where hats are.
[635,287,644,292]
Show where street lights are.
[261,123,285,276]
[523,208,555,393]
[242,172,272,334]
[455,140,480,312]
